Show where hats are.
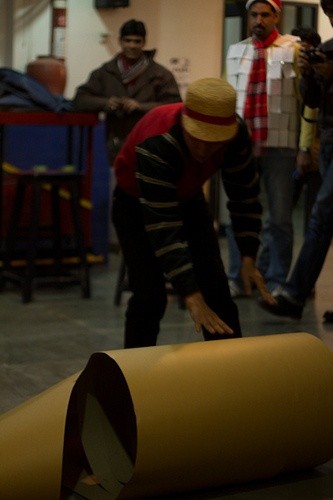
[245,0,283,14]
[181,78,240,144]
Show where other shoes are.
[257,290,305,321]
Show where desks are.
[0,110,100,276]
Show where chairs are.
[0,125,98,305]
[115,171,221,310]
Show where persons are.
[258,0,333,325]
[223,0,319,308]
[73,19,183,166]
[111,78,278,349]
[254,28,322,298]
